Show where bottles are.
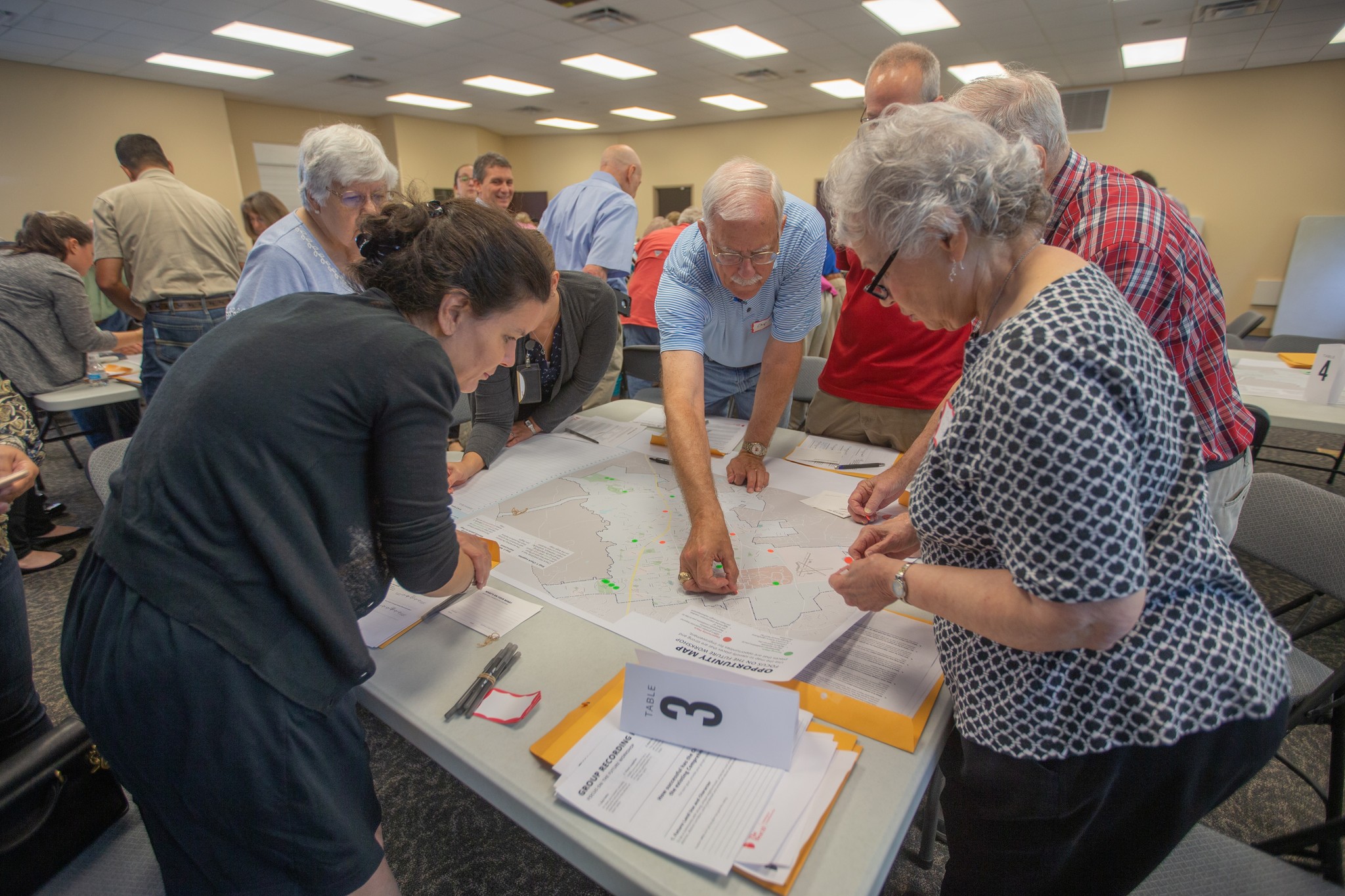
[86,356,108,386]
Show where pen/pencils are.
[835,463,885,469]
[649,457,671,465]
[421,593,461,621]
[444,642,522,720]
[564,428,599,443]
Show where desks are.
[349,398,950,896]
[27,352,143,470]
[1228,349,1345,436]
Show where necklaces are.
[532,305,561,355]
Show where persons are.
[61,198,552,896]
[240,191,291,246]
[454,151,541,229]
[445,228,617,493]
[0,375,54,765]
[537,144,642,410]
[847,69,1254,547]
[92,132,248,405]
[226,122,399,320]
[788,241,847,432]
[0,212,144,573]
[808,42,979,452]
[654,155,826,593]
[611,206,705,402]
[823,100,1293,896]
[1130,171,1156,188]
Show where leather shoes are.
[19,548,77,576]
[33,525,91,544]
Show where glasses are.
[861,105,878,128]
[711,235,780,265]
[863,247,900,300]
[324,185,394,209]
[457,175,478,183]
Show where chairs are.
[85,436,132,509]
[1226,310,1345,354]
[1126,403,1345,896]
[621,345,663,405]
[793,356,828,431]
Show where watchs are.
[892,562,918,605]
[522,418,536,435]
[742,442,767,456]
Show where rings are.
[510,432,514,438]
[755,469,763,472]
[678,572,693,583]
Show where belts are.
[825,273,845,280]
[146,293,232,312]
[1205,450,1246,473]
